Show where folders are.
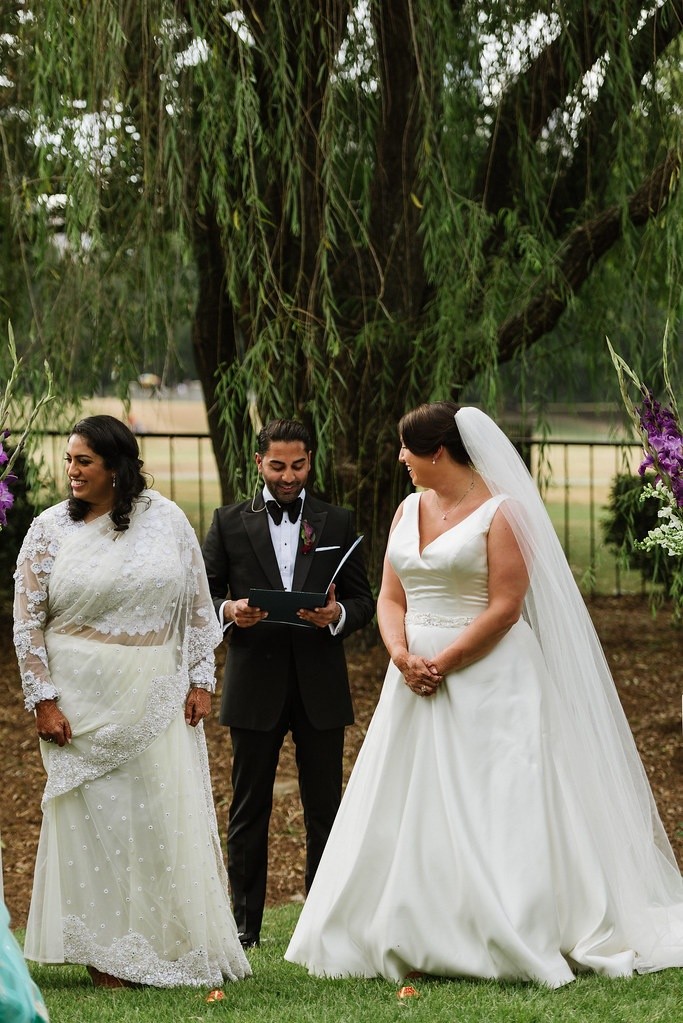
[247,535,365,631]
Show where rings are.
[45,738,53,744]
[420,685,427,693]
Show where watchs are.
[191,682,216,695]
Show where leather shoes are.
[235,926,260,947]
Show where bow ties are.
[266,497,302,525]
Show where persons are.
[200,420,372,951]
[375,401,683,985]
[13,414,224,989]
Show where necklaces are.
[432,468,475,519]
